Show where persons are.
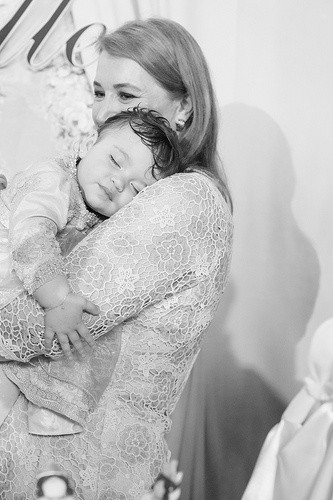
[0,101,182,365]
[1,18,235,499]
